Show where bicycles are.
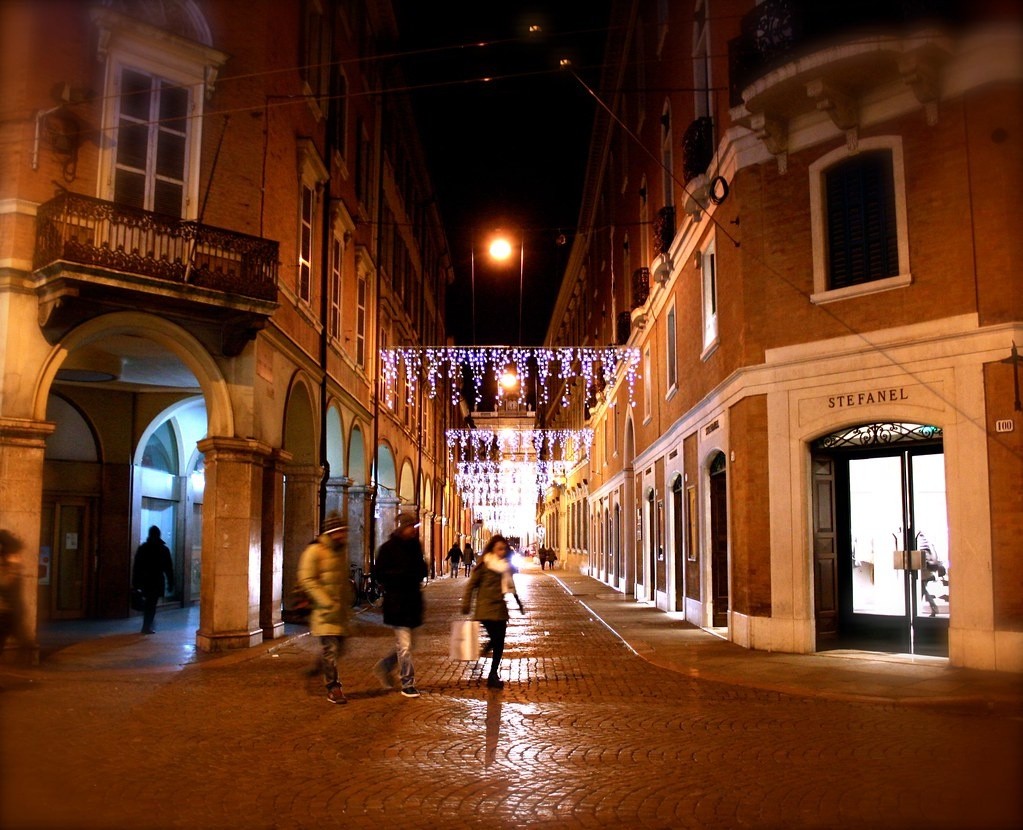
[420,575,429,589]
[350,562,387,609]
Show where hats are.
[323,509,349,535]
[394,511,417,530]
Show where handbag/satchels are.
[129,590,146,612]
[450,615,480,660]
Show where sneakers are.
[400,687,421,697]
[372,661,394,688]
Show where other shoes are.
[141,629,156,634]
[487,677,504,689]
[326,689,346,705]
[302,669,316,695]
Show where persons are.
[897,518,940,618]
[463,542,475,577]
[460,535,525,690]
[547,547,557,570]
[444,542,464,579]
[538,543,548,570]
[296,510,351,708]
[133,525,177,634]
[374,510,429,697]
[0,530,22,660]
